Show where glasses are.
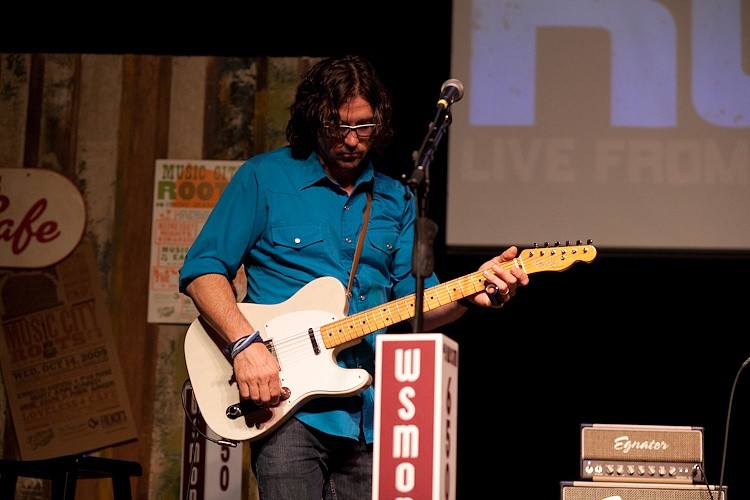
[324,123,380,140]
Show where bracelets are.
[227,331,264,357]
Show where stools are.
[0,457,142,500]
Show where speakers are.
[559,481,728,500]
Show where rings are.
[501,289,510,295]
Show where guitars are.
[183,238,597,443]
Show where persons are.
[179,54,529,500]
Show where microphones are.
[437,79,465,109]
